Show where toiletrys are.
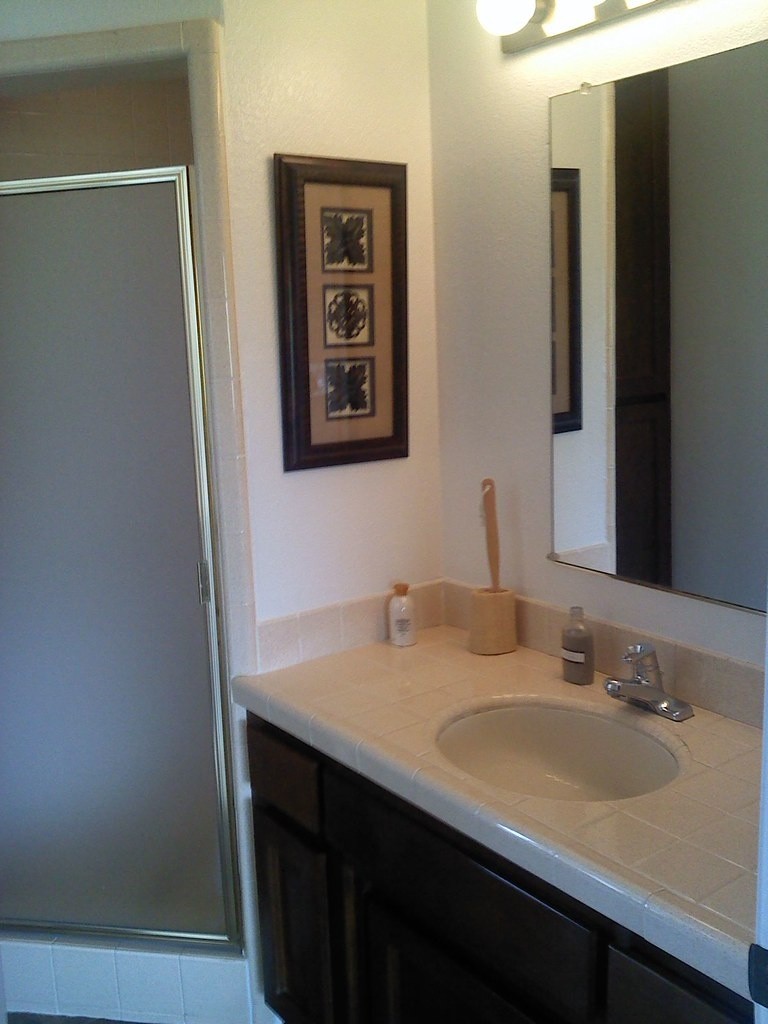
[559,604,596,686]
[387,583,417,647]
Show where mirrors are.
[548,38,768,619]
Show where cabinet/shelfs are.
[245,710,755,1024]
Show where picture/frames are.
[551,167,583,435]
[273,151,411,474]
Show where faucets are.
[601,641,665,717]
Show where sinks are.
[428,692,694,805]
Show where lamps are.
[475,0,668,55]
